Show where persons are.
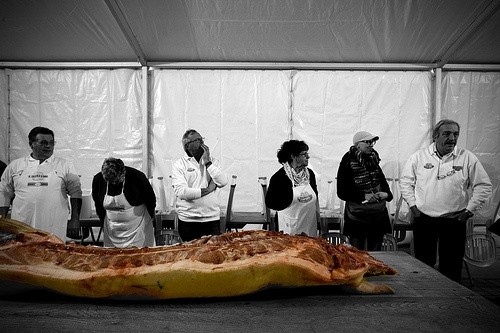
[92,158,156,249]
[337,131,393,251]
[265,140,322,238]
[172,130,228,241]
[0,127,82,240]
[400,119,493,282]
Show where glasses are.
[186,137,205,144]
[298,152,309,157]
[35,139,56,146]
[361,140,376,145]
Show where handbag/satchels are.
[347,197,386,217]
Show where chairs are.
[66,176,500,284]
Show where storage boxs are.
[67,188,92,219]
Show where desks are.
[81,212,491,227]
[0,251,500,333]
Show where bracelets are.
[465,210,473,217]
[205,161,212,166]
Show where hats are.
[353,131,379,143]
